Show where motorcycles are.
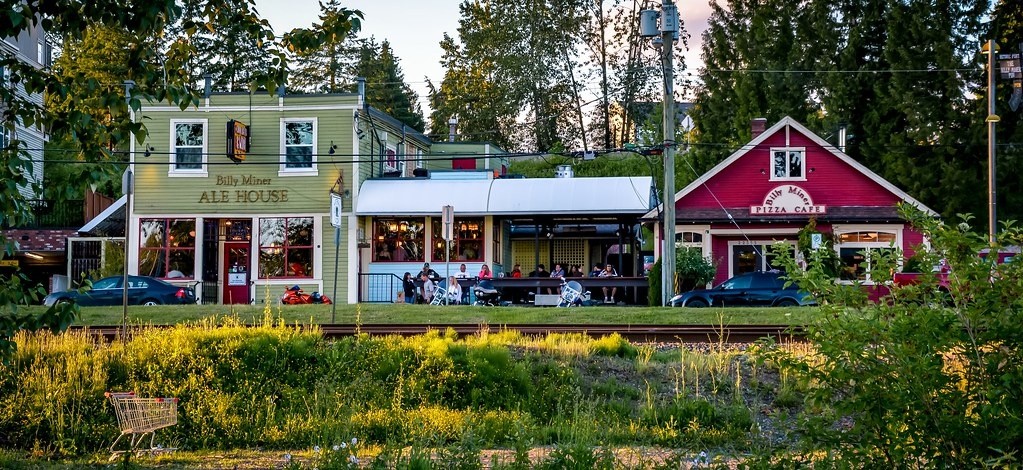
[554,273,615,307]
[425,281,457,308]
[281,286,332,305]
[469,276,514,308]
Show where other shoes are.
[611,298,614,303]
[604,298,607,302]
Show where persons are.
[479,264,491,279]
[510,264,521,278]
[403,262,462,307]
[528,262,618,304]
[454,265,469,304]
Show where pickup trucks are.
[886,249,1022,310]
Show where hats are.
[424,263,429,267]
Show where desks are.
[411,276,650,306]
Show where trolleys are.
[103,390,178,458]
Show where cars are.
[666,268,822,309]
[39,275,197,309]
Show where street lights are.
[640,1,680,308]
[979,38,1000,249]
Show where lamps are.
[144,142,155,157]
[328,141,338,155]
[761,168,765,174]
[809,168,815,174]
